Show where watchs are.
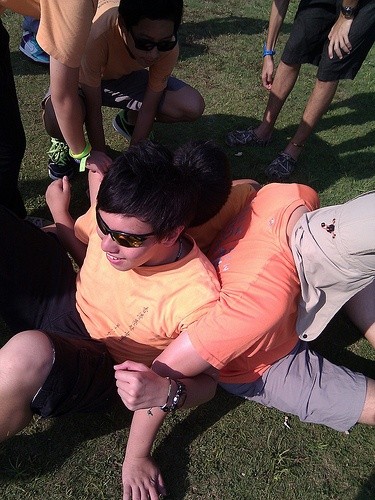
[341,4,357,18]
[162,379,187,412]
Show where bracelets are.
[262,37,275,57]
[159,377,172,409]
[67,141,92,172]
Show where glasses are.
[96,208,166,248]
[126,27,177,52]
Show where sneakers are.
[19,30,51,63]
[48,140,74,180]
[112,109,156,145]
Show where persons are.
[0,144,220,500]
[42,0,205,181]
[0,0,113,231]
[45,133,261,268]
[224,0,375,181]
[122,184,375,500]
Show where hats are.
[290,190,375,341]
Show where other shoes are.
[265,151,299,182]
[25,216,54,228]
[225,130,274,147]
